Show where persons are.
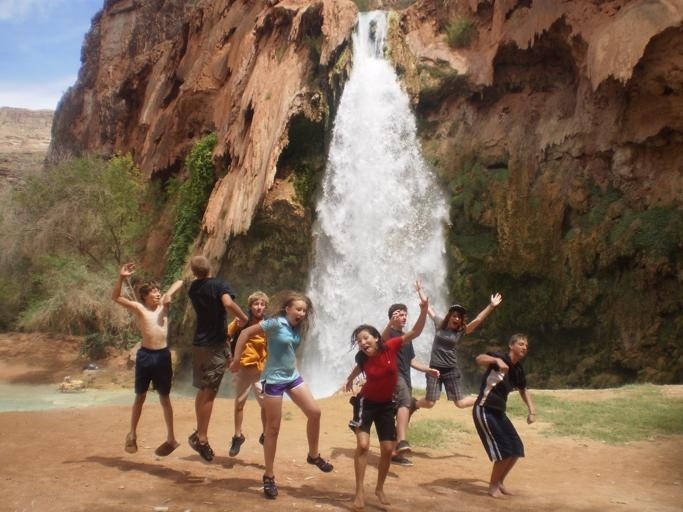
[109,262,183,458]
[409,278,502,422]
[379,303,439,464]
[344,297,428,509]
[473,332,536,500]
[227,290,274,457]
[189,256,248,461]
[229,293,335,497]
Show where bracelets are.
[428,367,433,375]
[387,323,391,328]
[530,413,535,415]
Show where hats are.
[449,305,468,315]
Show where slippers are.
[155,440,180,456]
[126,432,137,454]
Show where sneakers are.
[259,433,264,445]
[188,431,214,461]
[229,434,245,457]
[391,455,413,466]
[307,453,333,472]
[396,440,412,453]
[409,397,418,422]
[263,474,278,497]
